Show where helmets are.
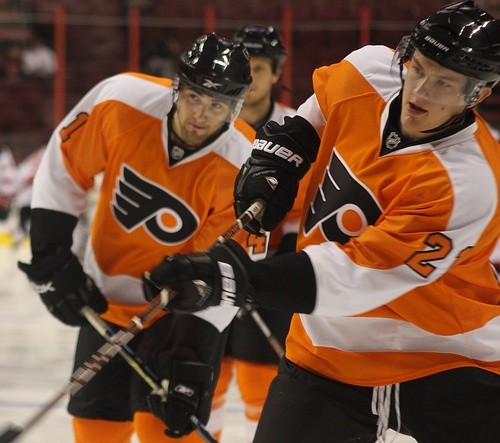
[177,32,252,103]
[237,24,285,56]
[397,0,500,81]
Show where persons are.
[201,20,302,443]
[22,32,260,443]
[150,0,500,443]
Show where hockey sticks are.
[243,302,287,359]
[79,302,219,443]
[1,198,265,443]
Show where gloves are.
[142,243,246,314]
[149,349,209,438]
[17,249,107,326]
[233,120,312,237]
